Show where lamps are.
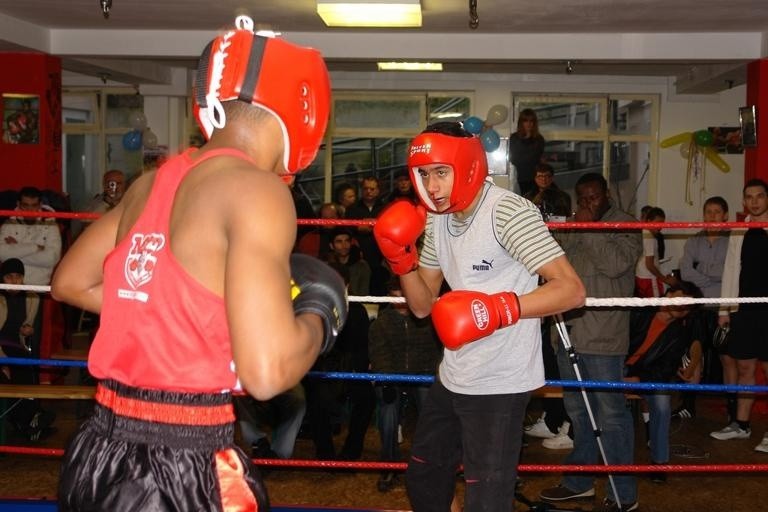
[317,1,425,27]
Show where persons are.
[51,32,345,512]
[2,188,61,337]
[540,174,643,511]
[635,206,667,297]
[318,203,345,230]
[86,171,125,214]
[0,260,58,448]
[331,183,358,206]
[710,178,768,455]
[523,324,577,451]
[626,280,704,482]
[510,109,545,199]
[345,175,384,221]
[672,196,736,421]
[523,165,571,216]
[373,122,587,512]
[386,172,416,203]
[327,227,363,278]
[301,302,371,473]
[366,286,444,492]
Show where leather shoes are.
[539,484,597,504]
[375,470,398,492]
[590,495,640,512]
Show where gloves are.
[373,198,430,277]
[291,252,350,357]
[431,287,520,350]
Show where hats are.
[2,256,26,276]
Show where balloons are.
[484,105,507,127]
[123,131,141,149]
[143,127,158,149]
[480,129,499,150]
[700,147,729,174]
[680,150,694,157]
[128,109,144,132]
[464,117,484,135]
[660,132,693,147]
[696,130,711,144]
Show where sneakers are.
[542,421,574,452]
[755,435,768,454]
[710,422,752,441]
[673,404,694,419]
[523,411,555,439]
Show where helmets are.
[406,131,489,214]
[191,28,331,174]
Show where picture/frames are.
[4,91,40,145]
